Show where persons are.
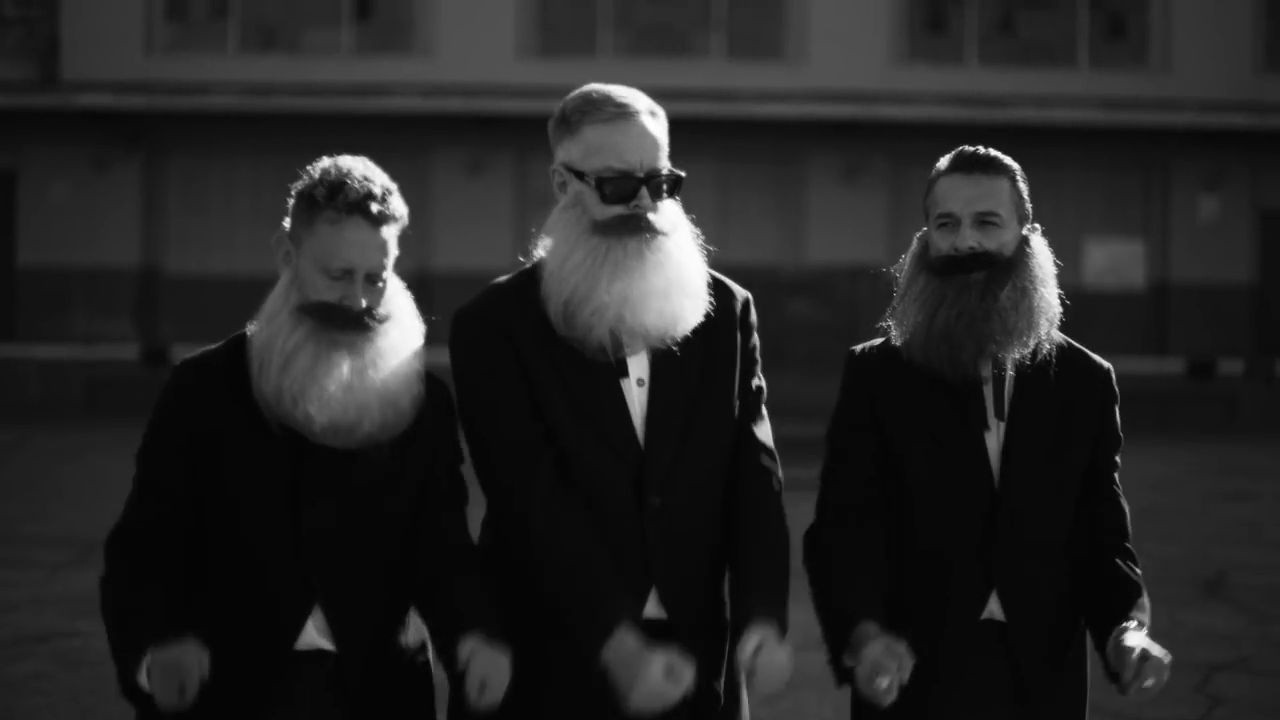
[447,78,802,720]
[799,145,1174,719]
[96,156,514,720]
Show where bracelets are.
[1105,620,1152,661]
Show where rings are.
[875,674,892,692]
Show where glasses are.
[567,166,684,204]
